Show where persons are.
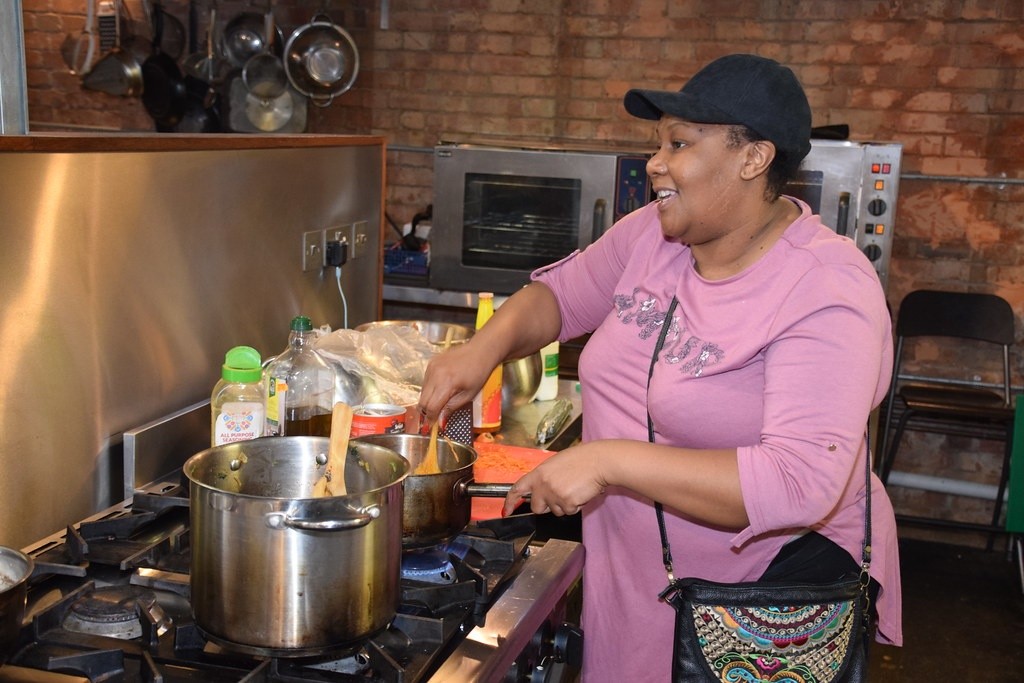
[417,53,905,683]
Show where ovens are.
[427,138,904,313]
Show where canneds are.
[346,403,407,438]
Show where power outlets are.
[303,221,371,271]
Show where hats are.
[624,54,812,171]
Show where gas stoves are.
[0,493,587,683]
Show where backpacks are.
[658,577,869,683]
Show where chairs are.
[876,289,1015,551]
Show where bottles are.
[536,341,560,401]
[265,314,337,438]
[472,290,504,427]
[209,345,270,448]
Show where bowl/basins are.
[281,22,358,107]
[502,352,541,410]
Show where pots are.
[350,433,537,549]
[0,544,35,648]
[181,435,412,651]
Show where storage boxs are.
[383,244,430,275]
